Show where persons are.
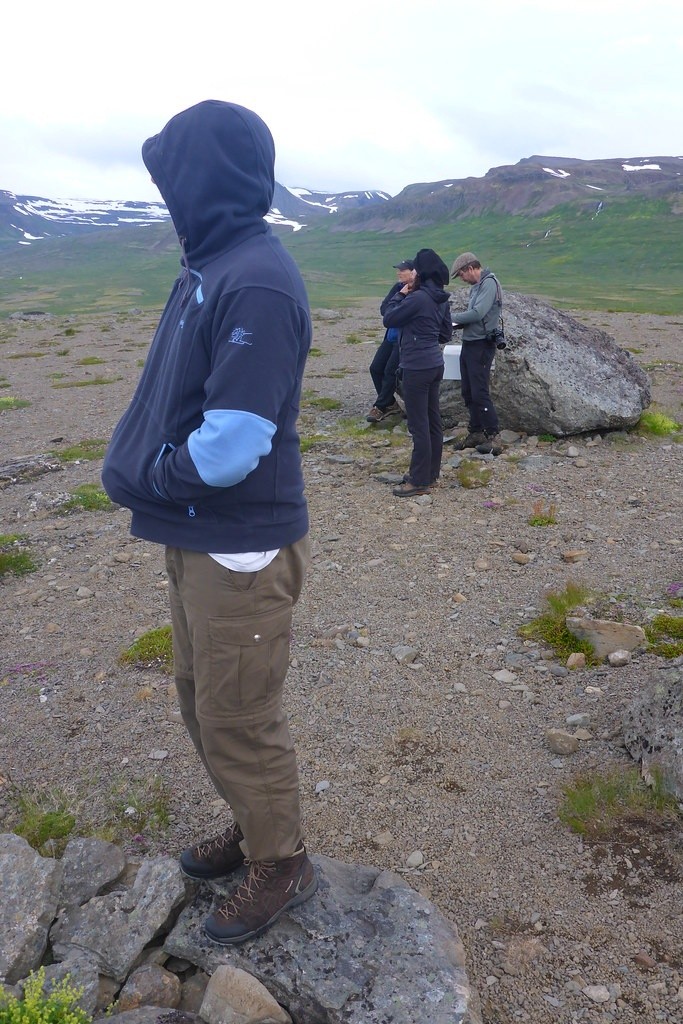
[100,103,320,947]
[368,247,505,494]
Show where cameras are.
[486,328,506,350]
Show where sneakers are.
[180,822,246,879]
[367,406,383,421]
[453,432,488,450]
[204,839,318,943]
[476,437,502,456]
[393,483,431,497]
[383,401,401,417]
[430,481,436,487]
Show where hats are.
[393,260,415,270]
[451,252,477,279]
